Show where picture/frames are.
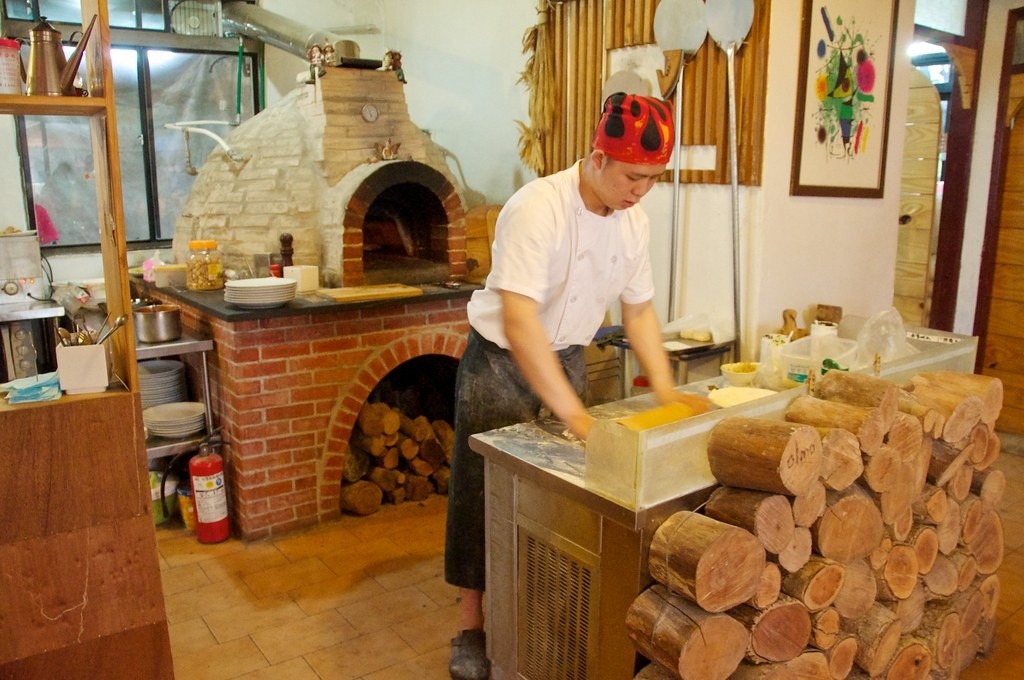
[785,0,900,199]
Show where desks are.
[608,341,731,399]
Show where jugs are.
[760,334,791,375]
[0,38,20,95]
[14,14,97,96]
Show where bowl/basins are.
[720,363,759,384]
[153,264,187,288]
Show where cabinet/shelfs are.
[69,311,220,459]
[0,0,175,680]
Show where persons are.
[443,93,712,680]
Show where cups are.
[283,265,319,294]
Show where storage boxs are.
[776,335,859,387]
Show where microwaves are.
[0,319,48,384]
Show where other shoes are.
[449,629,492,680]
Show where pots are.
[132,305,182,343]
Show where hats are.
[592,92,676,165]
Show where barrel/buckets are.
[149,471,179,526]
[177,479,197,532]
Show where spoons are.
[97,314,128,344]
[58,327,73,345]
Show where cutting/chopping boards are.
[316,283,424,302]
[468,376,722,530]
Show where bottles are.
[269,264,280,277]
[810,320,839,360]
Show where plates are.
[138,360,206,439]
[223,277,298,309]
[707,387,778,407]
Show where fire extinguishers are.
[160,426,231,543]
